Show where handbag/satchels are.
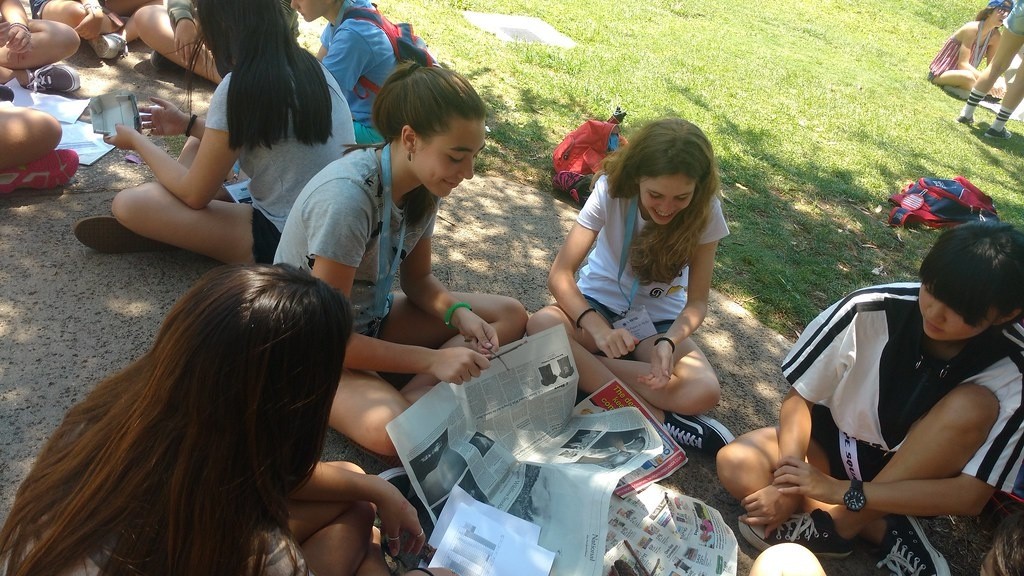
[552,106,629,175]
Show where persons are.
[273,61,528,457]
[748,512,1024,576]
[928,0,1024,139]
[0,0,357,264]
[526,118,736,454]
[290,0,401,149]
[0,262,461,576]
[716,218,1024,576]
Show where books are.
[386,323,738,576]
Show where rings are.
[416,528,424,537]
[386,533,401,542]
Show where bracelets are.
[81,2,102,9]
[843,478,866,513]
[403,567,434,576]
[654,336,675,353]
[444,301,473,330]
[186,114,197,137]
[8,22,31,37]
[576,308,596,328]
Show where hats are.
[987,0,1015,15]
[557,170,596,208]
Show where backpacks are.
[339,3,451,97]
[889,175,1001,229]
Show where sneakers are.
[739,508,853,560]
[664,410,735,453]
[879,513,949,576]
[20,66,80,94]
[985,126,1013,139]
[958,115,979,130]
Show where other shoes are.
[377,467,410,496]
[89,32,127,60]
[150,51,169,69]
[73,215,182,254]
[0,148,79,195]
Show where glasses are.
[994,7,1011,16]
